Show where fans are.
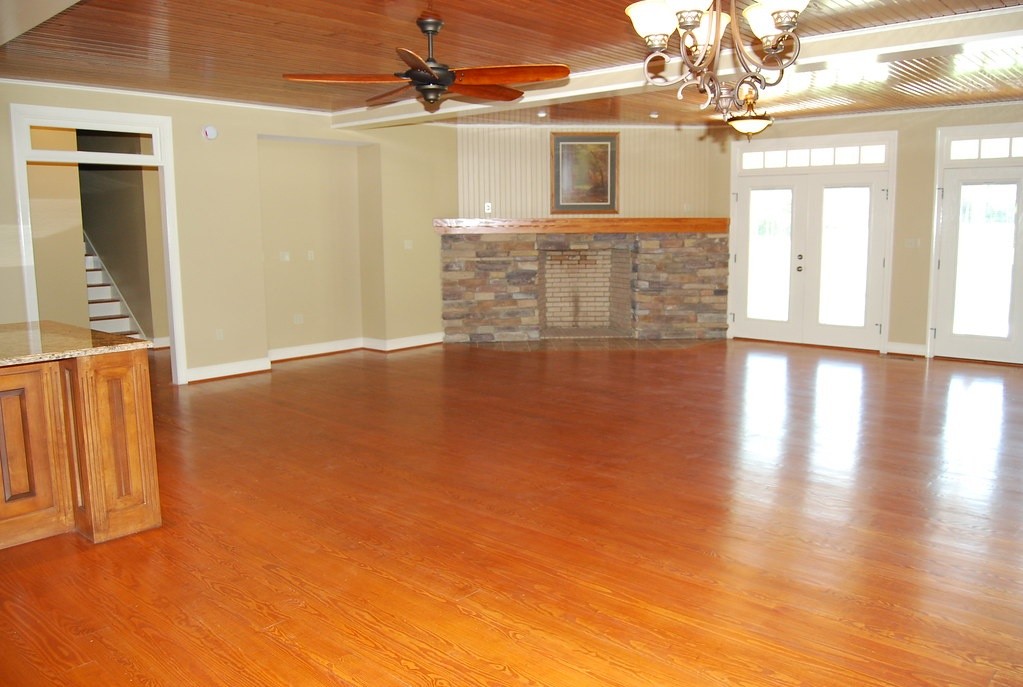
[282,18,570,106]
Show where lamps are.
[625,1,811,140]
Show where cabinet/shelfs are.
[0,321,163,551]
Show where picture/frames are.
[550,131,619,215]
